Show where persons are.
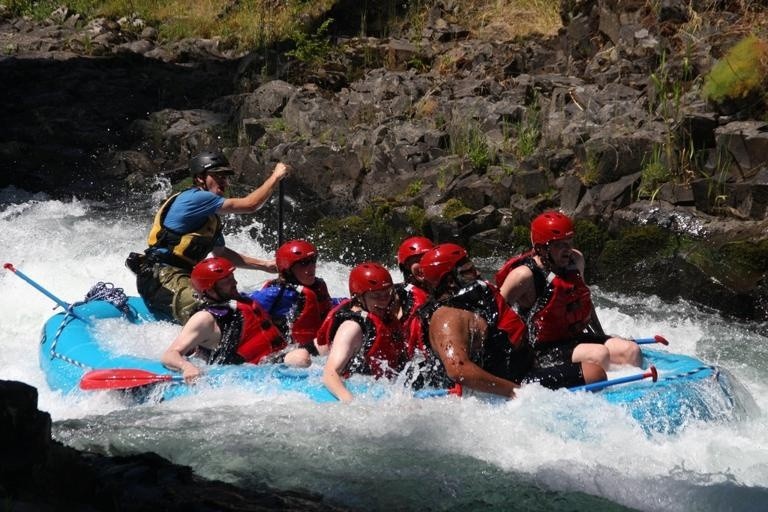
[398,234,439,359]
[159,257,312,385]
[312,262,410,402]
[137,148,295,326]
[417,244,608,399]
[252,239,349,355]
[495,210,642,386]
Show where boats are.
[40,295,738,455]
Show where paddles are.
[79,369,205,391]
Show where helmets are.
[396,235,435,269]
[418,243,469,289]
[529,210,576,250]
[190,255,238,294]
[348,261,394,298]
[191,149,237,180]
[274,238,320,273]
